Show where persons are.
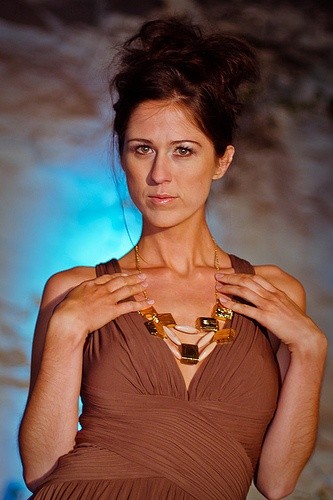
[15,13,327,500]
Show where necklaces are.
[131,244,238,365]
